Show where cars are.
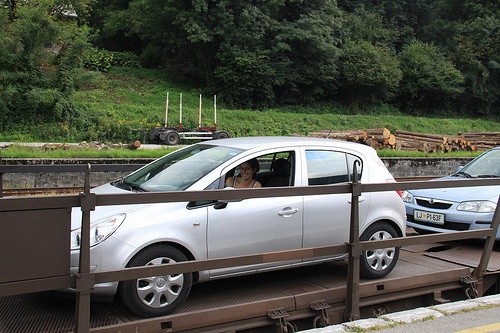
[402,146,500,241]
[66,115,408,317]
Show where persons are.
[224,158,261,189]
